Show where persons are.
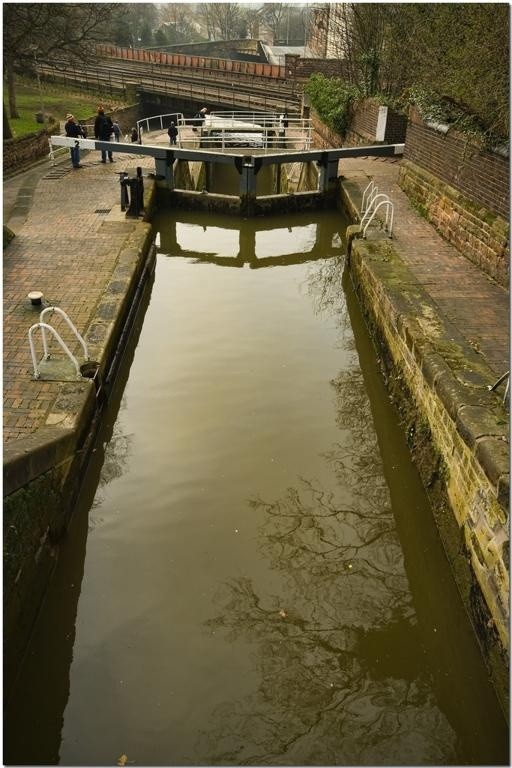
[167,121,179,146]
[65,106,143,169]
[192,108,207,131]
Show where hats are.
[66,113,73,121]
[98,104,105,112]
